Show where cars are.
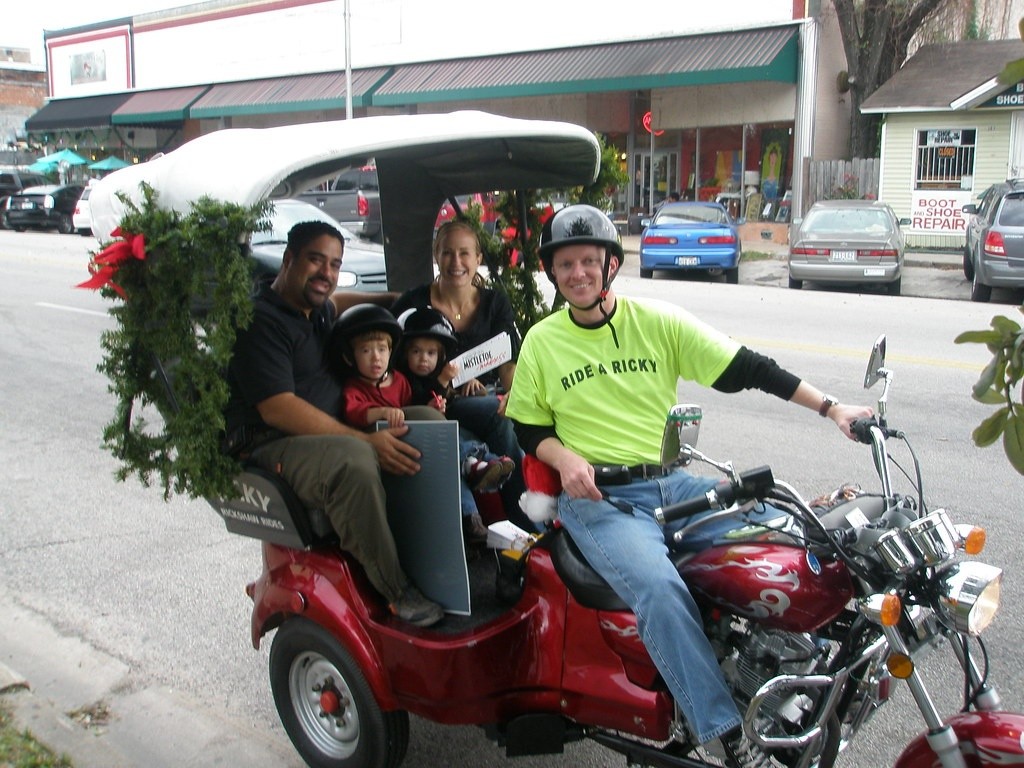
[640,200,746,283]
[6,185,94,236]
[250,166,388,295]
[789,199,911,296]
[433,194,614,264]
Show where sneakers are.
[703,724,787,768]
[493,571,522,605]
[385,584,443,626]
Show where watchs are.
[818,395,839,417]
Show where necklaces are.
[455,314,461,321]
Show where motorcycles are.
[92,109,1024,768]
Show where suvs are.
[962,177,1024,302]
[0,172,57,231]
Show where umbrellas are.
[88,155,130,171]
[36,148,90,167]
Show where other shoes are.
[489,457,515,489]
[466,460,503,493]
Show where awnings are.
[189,66,394,119]
[111,85,221,123]
[25,92,183,132]
[372,26,799,106]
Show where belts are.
[627,456,689,476]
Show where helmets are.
[396,306,456,351]
[333,303,402,353]
[535,204,624,267]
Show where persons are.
[389,221,546,533]
[215,220,445,626]
[505,204,877,768]
[330,303,490,542]
[394,304,516,494]
